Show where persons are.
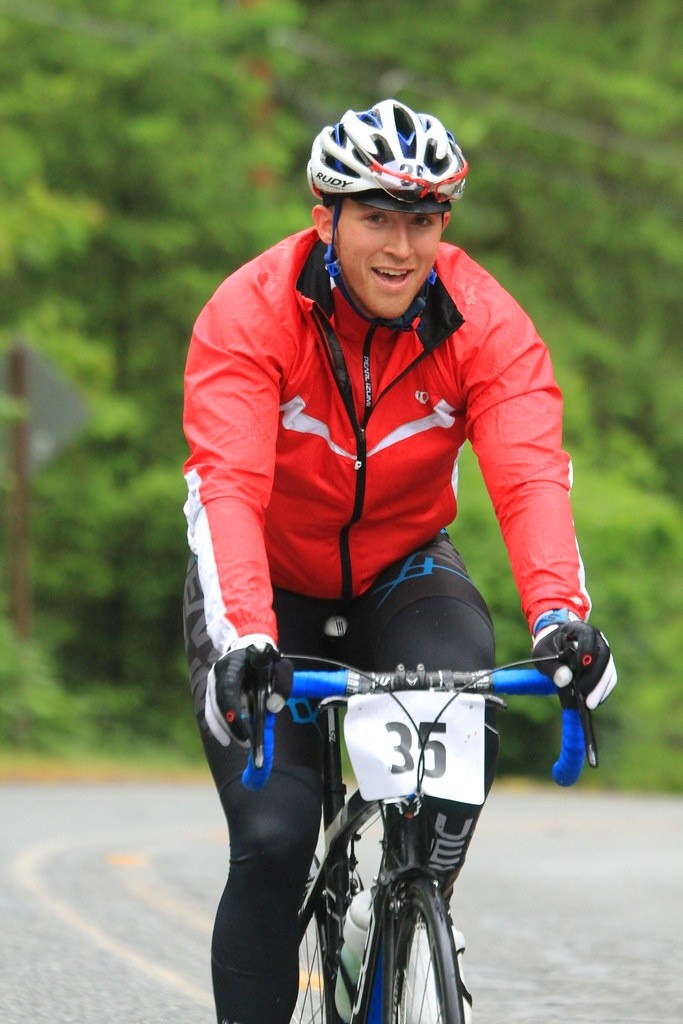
[181,100,616,1024]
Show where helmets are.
[309,99,467,215]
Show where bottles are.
[333,886,377,1023]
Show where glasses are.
[344,127,468,204]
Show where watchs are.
[535,608,580,633]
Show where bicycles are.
[241,637,602,1024]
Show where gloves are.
[530,623,618,709]
[204,641,298,751]
